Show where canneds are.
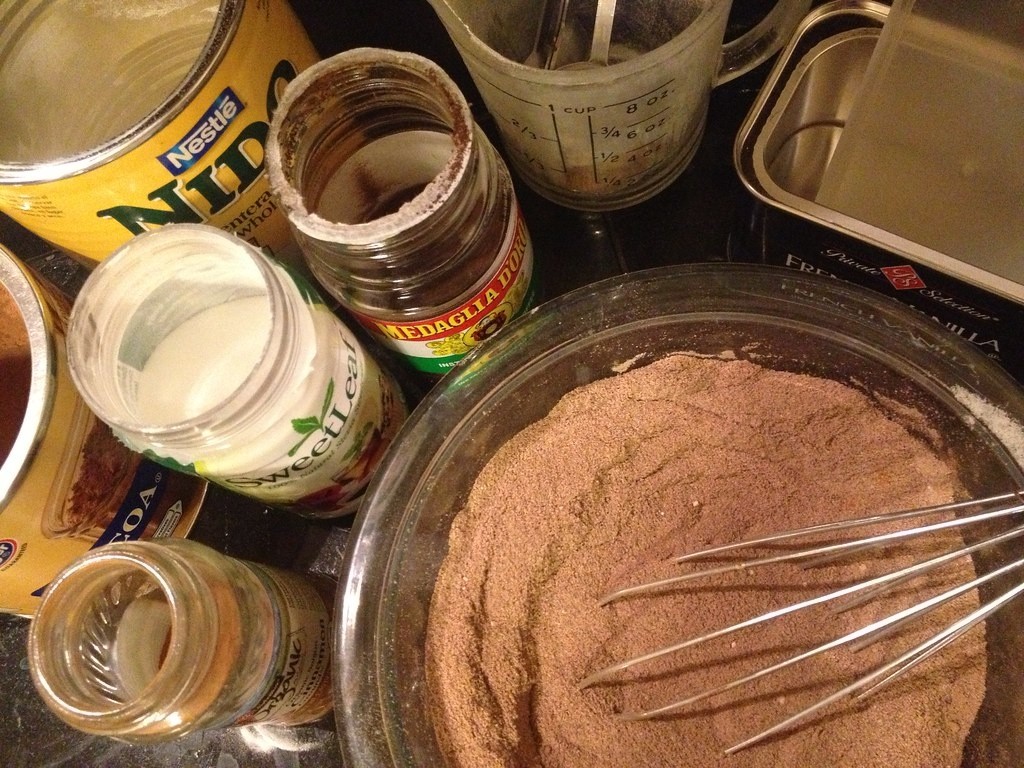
[0,0,730,741]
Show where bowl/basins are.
[334,265,1024,768]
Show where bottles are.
[68,224,399,517]
[264,47,540,381]
[29,547,331,745]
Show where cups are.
[428,0,806,210]
